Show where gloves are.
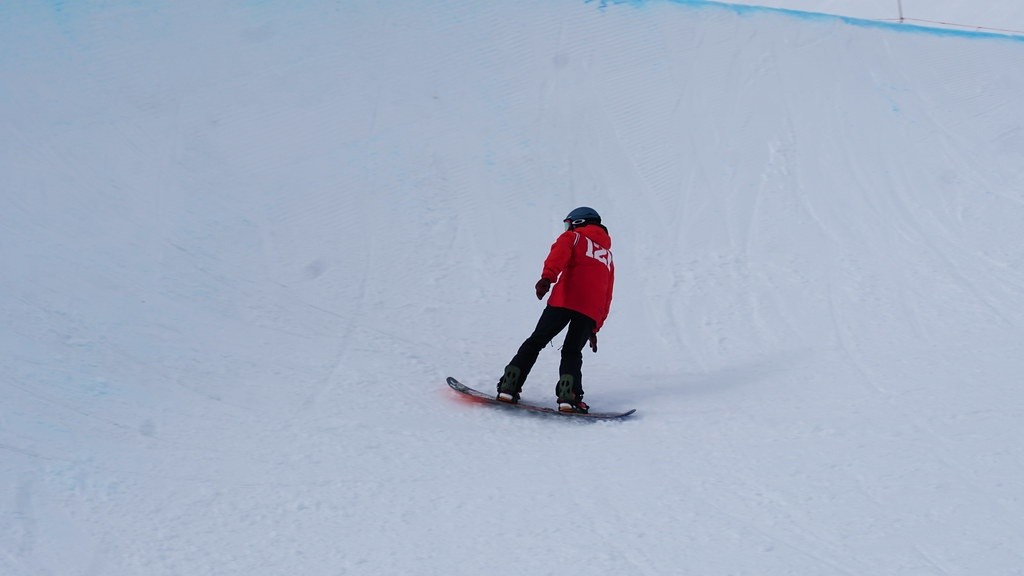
[589,332,599,353]
[535,278,550,300]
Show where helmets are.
[564,206,602,226]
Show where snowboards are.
[445,373,640,420]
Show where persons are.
[495,207,614,412]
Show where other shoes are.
[558,399,590,413]
[495,388,521,403]
